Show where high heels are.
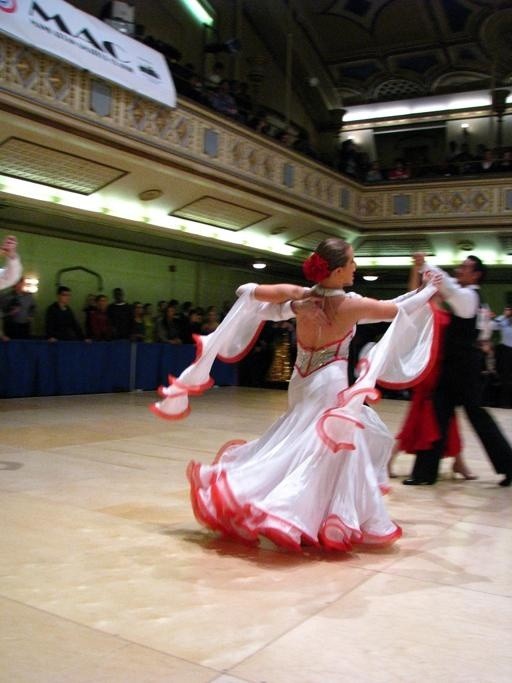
[453,463,479,480]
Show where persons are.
[147,238,443,555]
[402,249,512,486]
[441,141,512,176]
[266,270,434,407]
[474,306,512,407]
[341,140,410,180]
[264,320,295,390]
[170,51,317,159]
[0,235,233,344]
[387,252,478,480]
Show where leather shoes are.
[500,472,512,486]
[403,477,436,485]
